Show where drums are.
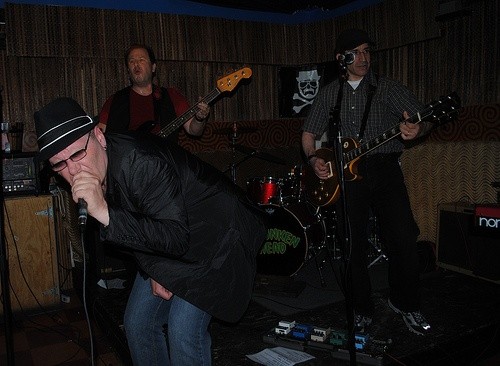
[245,176,295,206]
[248,196,327,277]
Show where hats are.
[333,29,375,52]
[33,96,99,164]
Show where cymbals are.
[211,126,255,134]
[230,144,286,165]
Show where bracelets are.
[308,155,316,160]
[195,115,205,122]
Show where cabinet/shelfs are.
[0,194,60,312]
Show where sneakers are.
[387,298,432,337]
[355,310,374,333]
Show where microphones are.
[335,52,357,63]
[77,198,89,225]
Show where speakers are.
[435,201,500,283]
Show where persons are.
[34,96,269,366]
[98,44,211,156]
[301,29,434,337]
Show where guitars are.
[303,91,462,208]
[137,68,252,139]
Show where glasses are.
[347,47,369,55]
[42,132,92,172]
